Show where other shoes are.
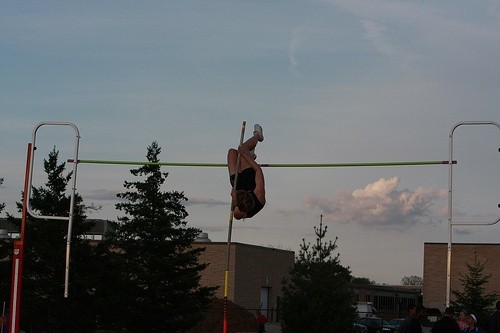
[253,124,264,142]
[249,147,257,160]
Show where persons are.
[255,309,267,333]
[488,301,500,333]
[226,123,266,221]
[397,303,423,333]
[430,306,484,333]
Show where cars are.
[349,316,406,333]
